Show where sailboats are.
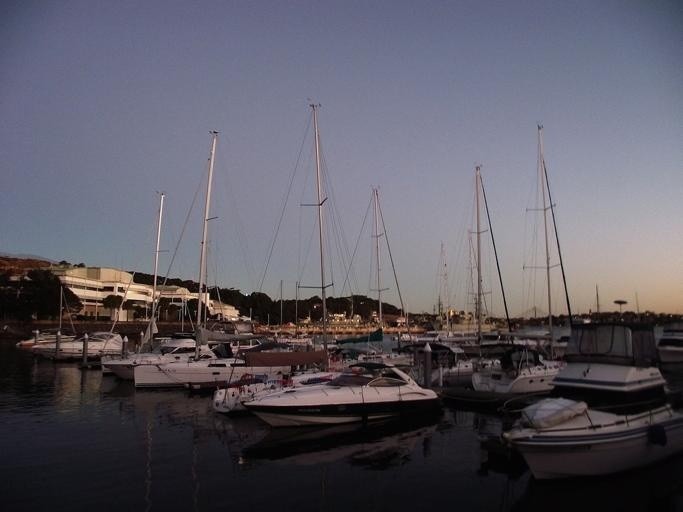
[209,101,448,432]
[13,286,78,354]
[100,127,292,392]
[35,270,136,364]
[332,124,579,402]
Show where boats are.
[499,320,683,487]
